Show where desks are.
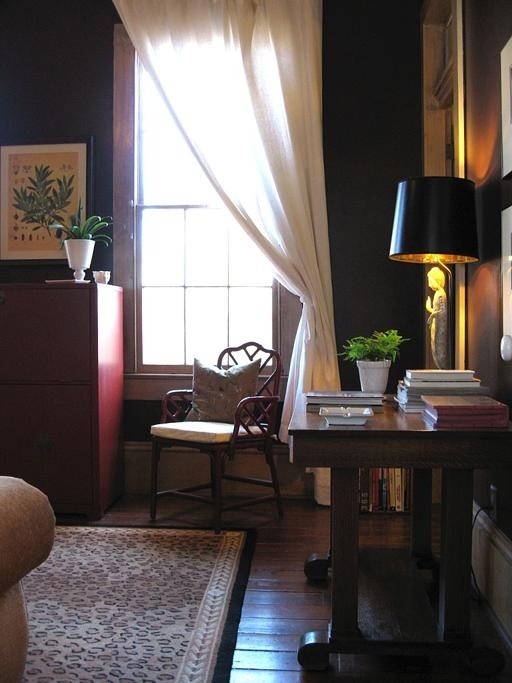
[288,393,512,672]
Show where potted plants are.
[43,193,112,285]
[338,329,414,394]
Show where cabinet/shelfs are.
[0,282,125,520]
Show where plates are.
[319,407,373,424]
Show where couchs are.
[1,475,58,682]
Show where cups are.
[93,270,111,285]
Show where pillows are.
[183,357,261,425]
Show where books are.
[394,370,509,428]
[306,391,385,414]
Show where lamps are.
[389,176,478,370]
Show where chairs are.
[150,341,282,536]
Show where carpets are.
[20,523,256,682]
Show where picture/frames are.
[0,134,95,269]
